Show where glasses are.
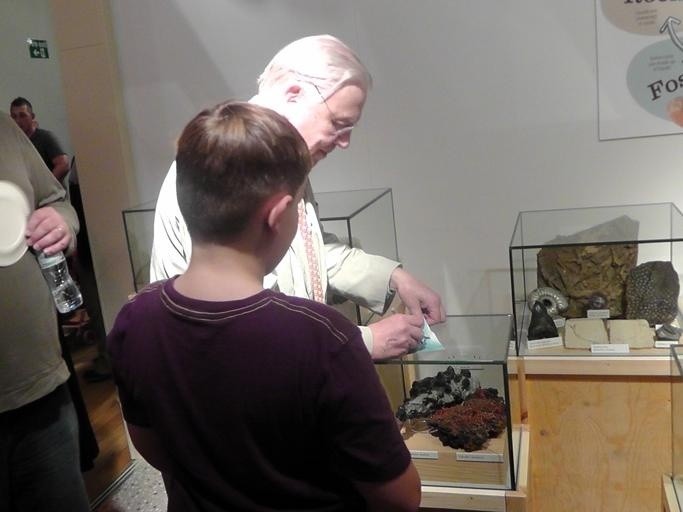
[302,80,354,145]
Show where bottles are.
[34,247,83,313]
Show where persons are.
[8,98,70,181]
[106,100,423,512]
[150,35,447,365]
[1,111,90,512]
